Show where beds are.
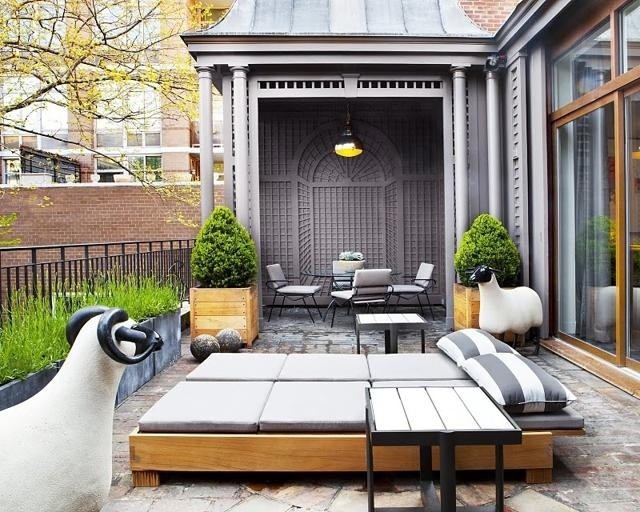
[127,345,587,490]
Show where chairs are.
[266,259,439,329]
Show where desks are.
[364,385,523,510]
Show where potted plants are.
[453,212,522,345]
[189,203,262,350]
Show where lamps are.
[334,101,365,158]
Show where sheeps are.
[0,305,165,512]
[464,265,544,356]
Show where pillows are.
[436,327,579,416]
